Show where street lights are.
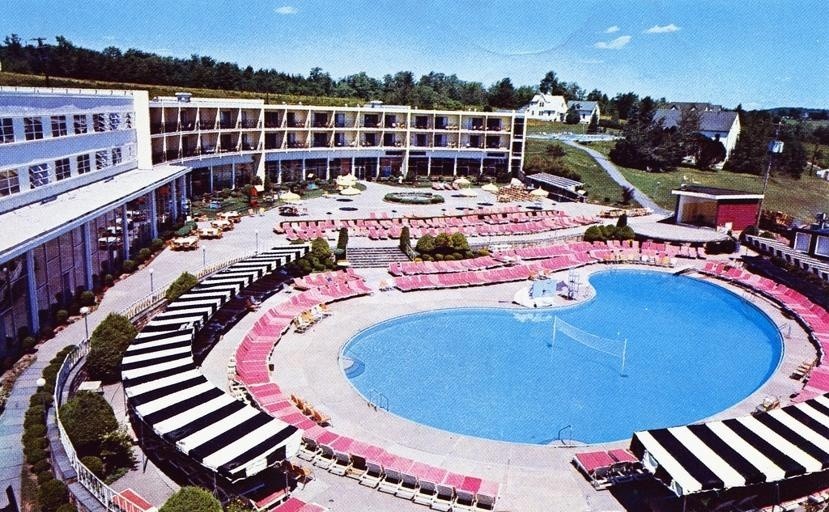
[202,244,206,267]
[149,268,154,292]
[37,376,48,421]
[79,306,91,339]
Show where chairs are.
[702,258,829,408]
[230,267,374,368]
[573,450,652,491]
[280,202,603,242]
[229,368,503,512]
[390,240,707,292]
